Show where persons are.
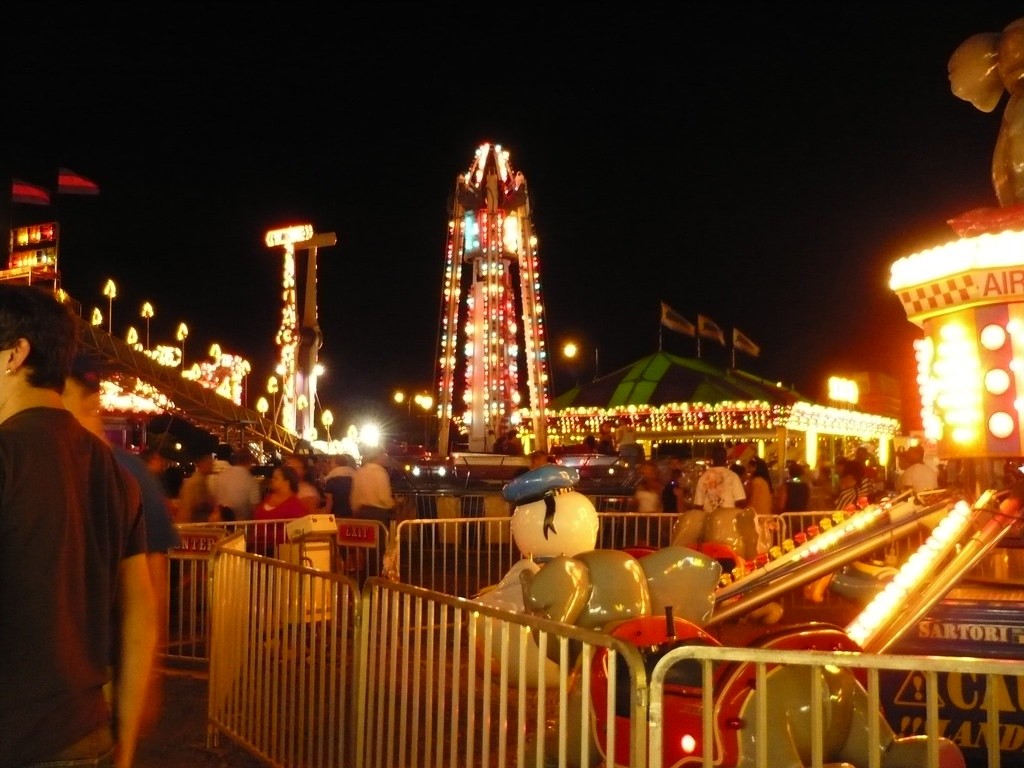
[583,415,636,455]
[778,463,810,512]
[486,428,522,456]
[0,283,180,768]
[636,445,772,515]
[825,445,947,510]
[141,442,396,570]
